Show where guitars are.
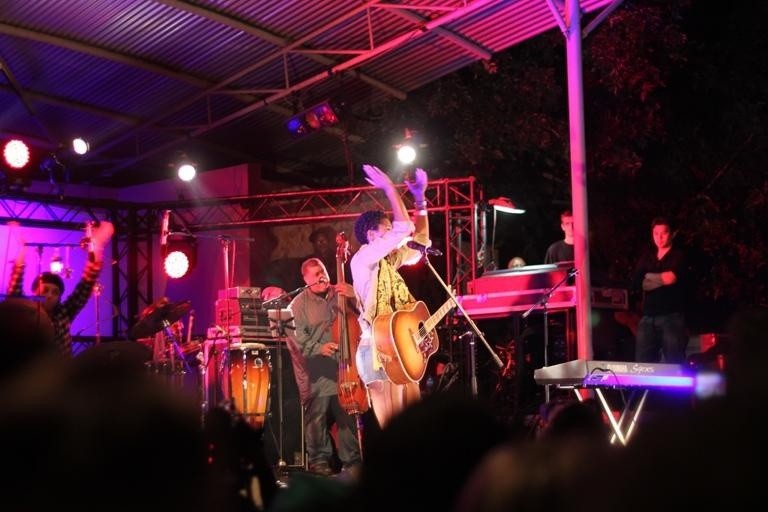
[372,288,458,385]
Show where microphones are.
[85,222,94,262]
[406,241,442,257]
[159,211,169,258]
[319,278,328,284]
[553,267,578,293]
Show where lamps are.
[71,136,96,155]
[284,94,351,143]
[170,149,196,183]
[163,231,198,277]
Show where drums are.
[165,340,201,368]
[217,342,273,436]
[144,358,172,378]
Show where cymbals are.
[130,299,192,338]
[69,341,152,378]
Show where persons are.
[0,163,767,512]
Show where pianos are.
[534,359,727,390]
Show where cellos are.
[331,231,372,415]
